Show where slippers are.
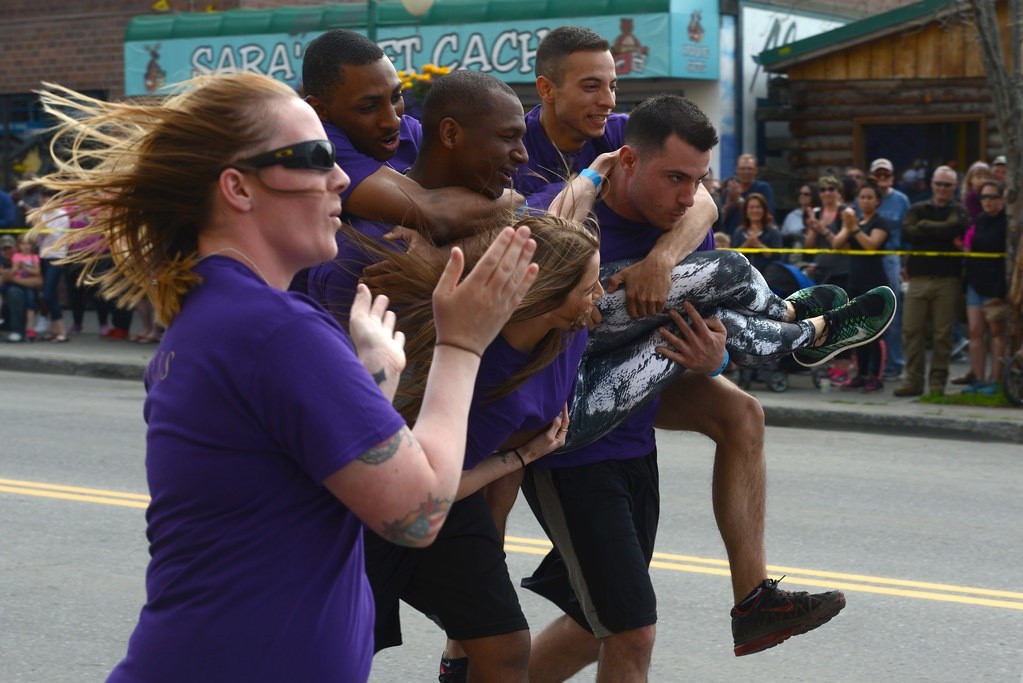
[126,335,160,344]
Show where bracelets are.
[578,166,602,187]
[434,340,482,359]
[512,446,525,467]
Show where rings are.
[559,428,568,433]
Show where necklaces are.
[550,136,584,188]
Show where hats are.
[992,155,1008,166]
[869,158,894,172]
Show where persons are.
[526,97,754,683]
[287,31,422,335]
[507,25,847,656]
[325,199,898,508]
[356,69,532,683]
[28,70,541,683]
[0,154,1023,403]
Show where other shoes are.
[26,328,37,342]
[840,376,885,394]
[36,331,70,344]
[820,367,852,387]
[951,370,975,385]
[101,328,129,341]
[893,382,924,396]
[70,323,83,334]
[7,332,24,343]
[961,379,998,395]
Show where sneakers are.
[785,284,849,322]
[792,286,897,367]
[730,575,846,656]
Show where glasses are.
[870,172,895,179]
[980,194,1002,199]
[819,186,835,192]
[797,193,811,196]
[213,139,336,181]
[932,181,957,188]
